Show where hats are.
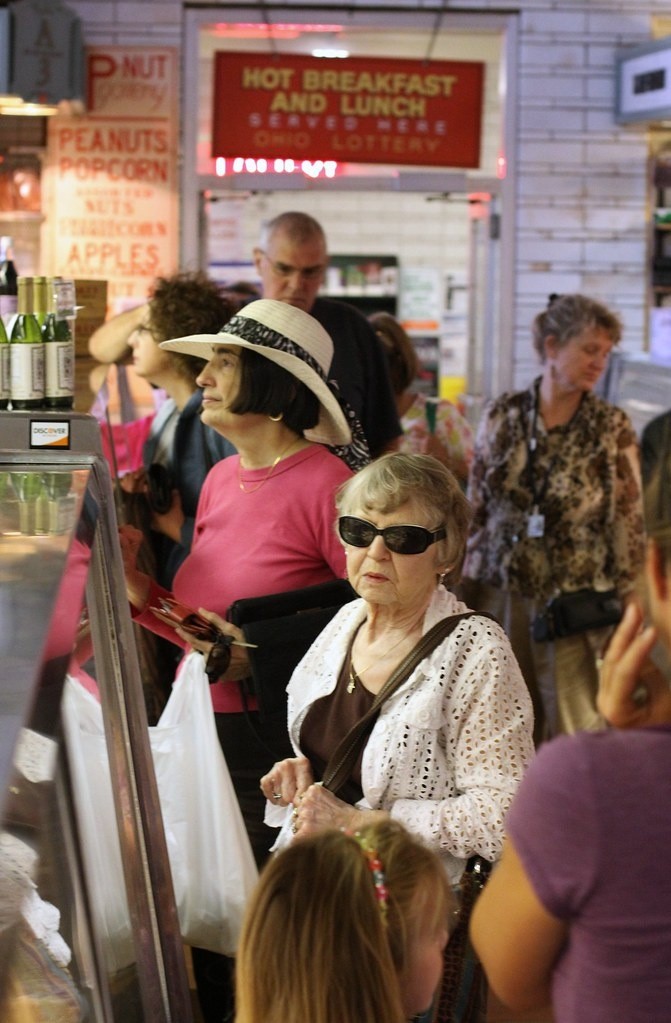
[158,299,370,475]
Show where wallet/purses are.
[150,597,218,655]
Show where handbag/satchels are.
[146,650,263,960]
[531,587,622,642]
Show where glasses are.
[338,516,446,555]
[136,323,155,335]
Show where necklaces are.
[238,435,301,494]
[346,616,423,693]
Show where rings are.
[595,658,603,669]
[291,808,298,819]
[298,794,304,801]
[290,823,298,834]
[272,793,282,799]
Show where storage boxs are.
[72,279,108,357]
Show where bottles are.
[1,276,73,411]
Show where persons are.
[468,410,671,1023]
[233,819,454,1023]
[27,212,671,1023]
